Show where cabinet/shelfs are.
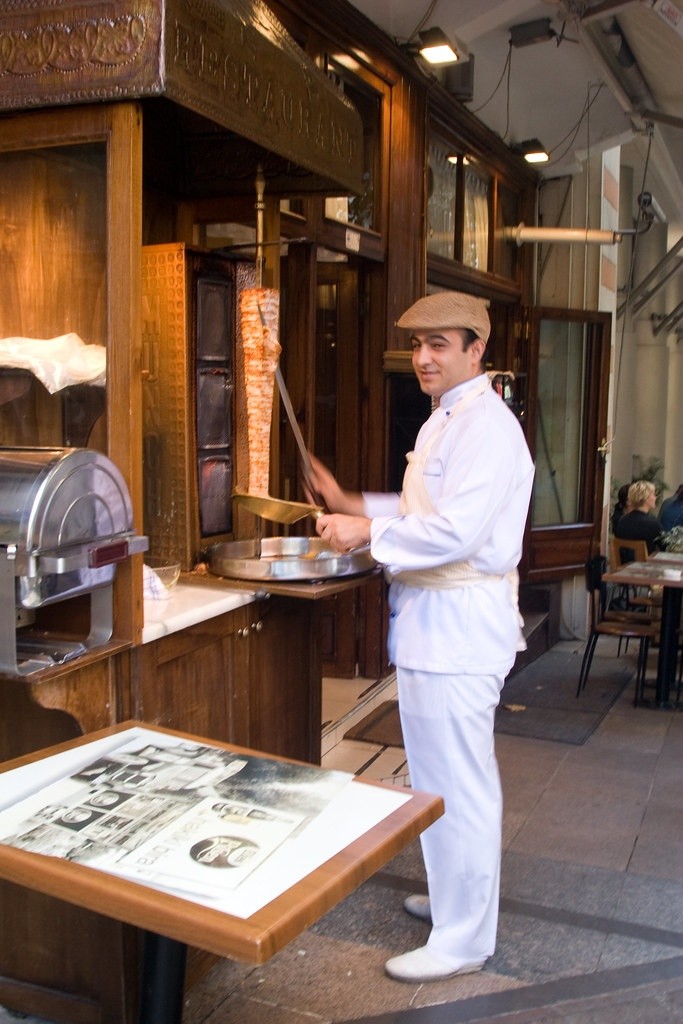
[141,240,238,575]
[118,594,323,767]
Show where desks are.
[0,716,446,1024]
[646,550,683,564]
[601,561,683,710]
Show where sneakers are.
[385,944,485,982]
[404,894,433,924]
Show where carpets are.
[495,634,653,746]
[341,700,405,747]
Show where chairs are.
[576,536,683,714]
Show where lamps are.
[400,25,459,66]
[508,138,551,164]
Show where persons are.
[612,480,683,566]
[303,293,535,984]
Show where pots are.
[206,536,379,580]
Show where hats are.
[397,291,491,345]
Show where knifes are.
[258,304,355,554]
